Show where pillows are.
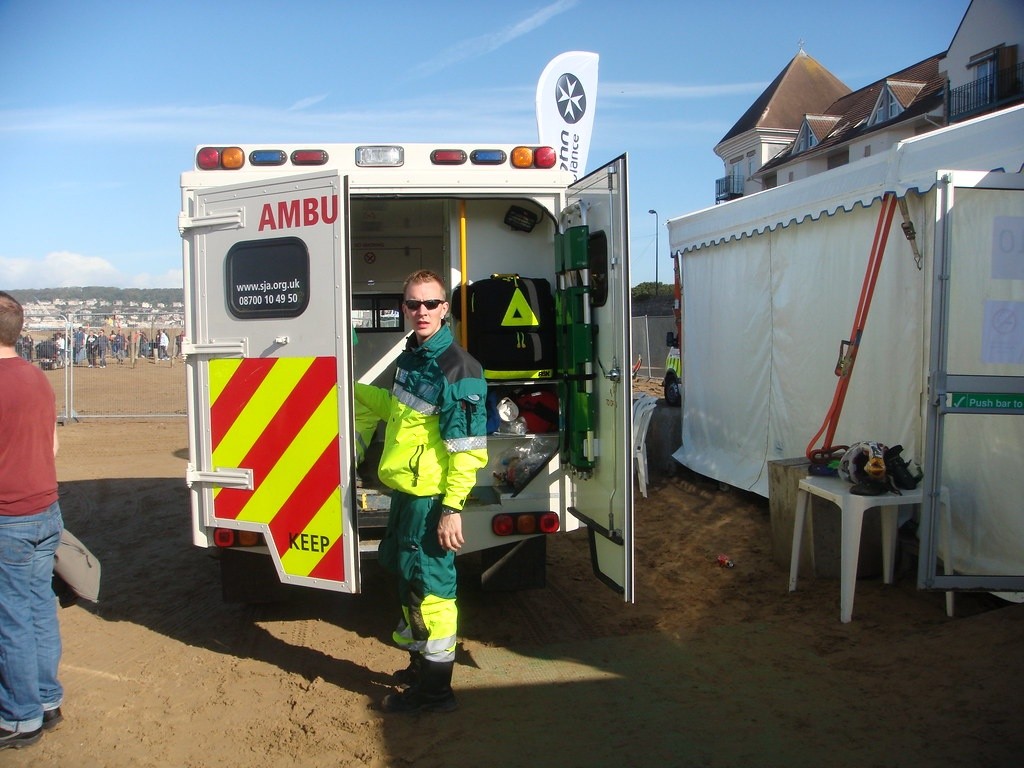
[54,528,101,603]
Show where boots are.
[380,650,457,712]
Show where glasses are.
[404,300,445,310]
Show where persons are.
[0,291,64,752]
[353,269,489,712]
[14,325,183,370]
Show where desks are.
[789,476,953,623]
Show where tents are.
[670,105,1024,602]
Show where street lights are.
[648,209,658,294]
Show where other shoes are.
[162,357,169,360]
[0,725,43,750]
[43,707,62,729]
[88,365,96,368]
[100,366,105,369]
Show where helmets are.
[838,440,890,491]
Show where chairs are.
[631,396,660,499]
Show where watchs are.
[441,508,454,514]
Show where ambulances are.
[177,140,636,607]
